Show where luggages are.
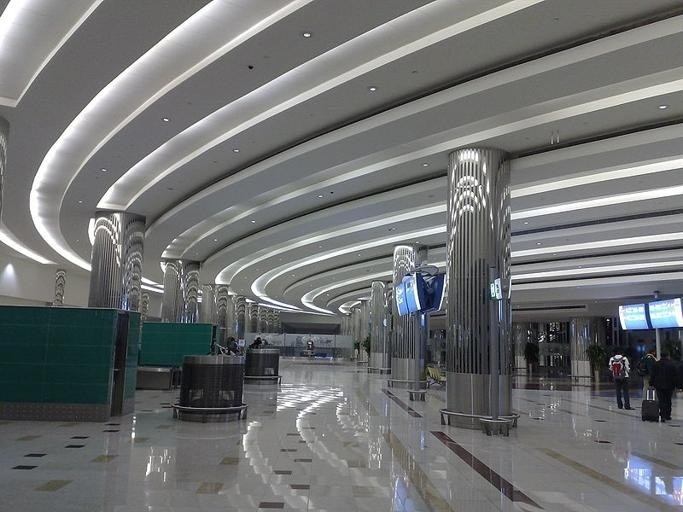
[642,389,660,422]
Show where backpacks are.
[611,356,625,380]
[637,355,652,376]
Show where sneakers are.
[661,417,671,422]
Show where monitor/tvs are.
[617,296,683,331]
[394,271,447,317]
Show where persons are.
[609,352,637,411]
[649,351,679,423]
[637,349,658,401]
